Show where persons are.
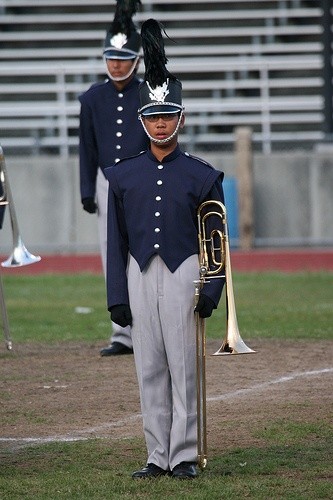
[107,79,225,478]
[78,27,146,355]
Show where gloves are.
[81,197,98,213]
[196,294,214,318]
[111,304,132,327]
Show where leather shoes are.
[172,461,197,478]
[99,342,133,356]
[131,463,167,479]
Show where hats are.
[138,18,185,116]
[103,0,143,60]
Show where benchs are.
[0,0,325,155]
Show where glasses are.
[140,113,178,122]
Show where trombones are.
[0,145,42,352]
[192,199,258,472]
